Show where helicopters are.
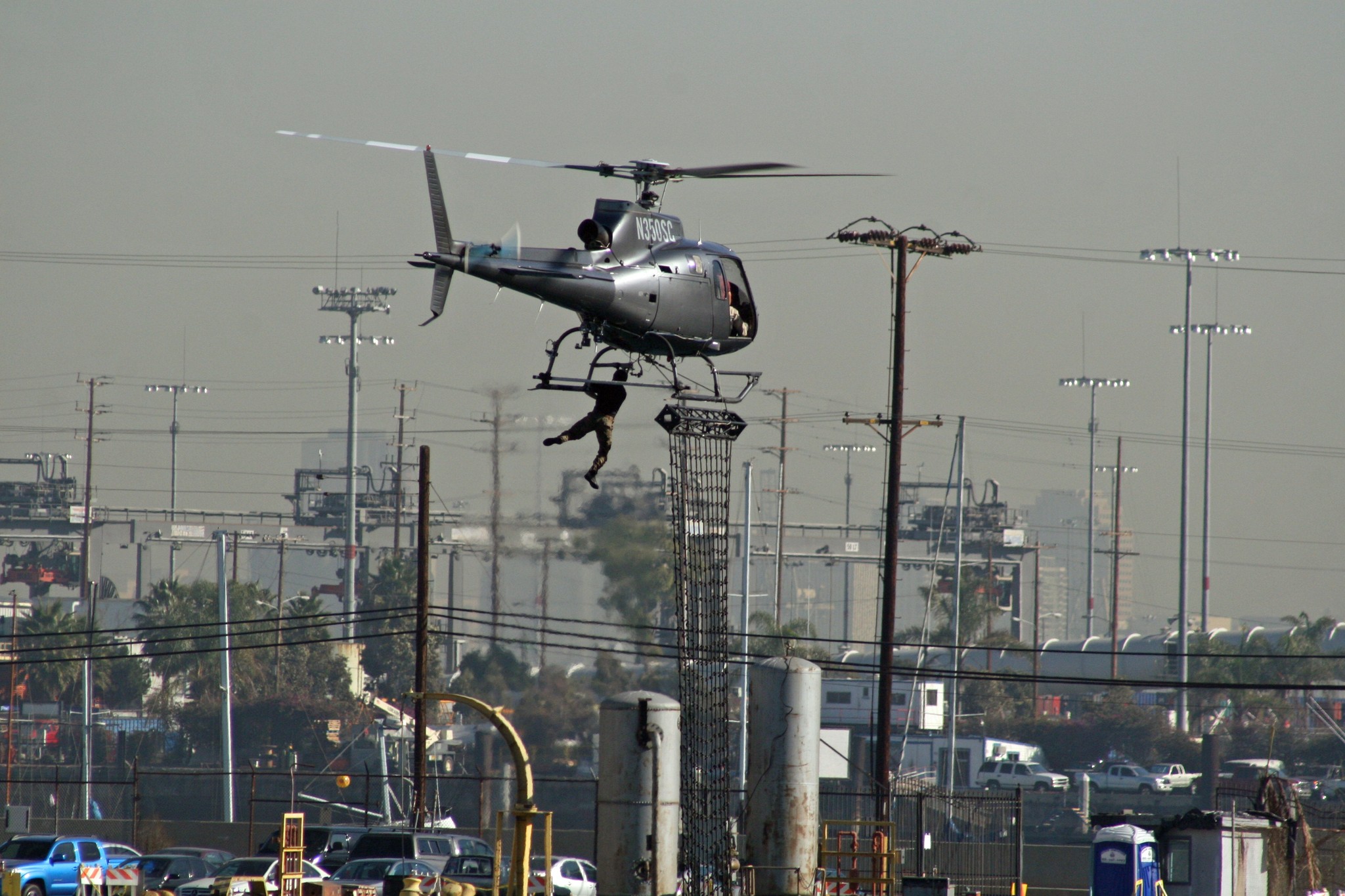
[267,125,901,407]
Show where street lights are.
[1095,465,1139,639]
[1058,377,1131,641]
[143,381,208,588]
[253,595,312,697]
[309,283,401,645]
[821,440,879,651]
[1138,246,1240,743]
[1167,322,1254,631]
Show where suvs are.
[974,759,1070,793]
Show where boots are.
[543,436,564,446]
[584,470,599,490]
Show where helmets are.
[612,370,628,382]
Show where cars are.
[1286,763,1345,805]
[1218,758,1285,785]
[0,805,597,896]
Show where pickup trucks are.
[1073,764,1174,796]
[1149,762,1202,795]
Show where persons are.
[543,369,632,485]
